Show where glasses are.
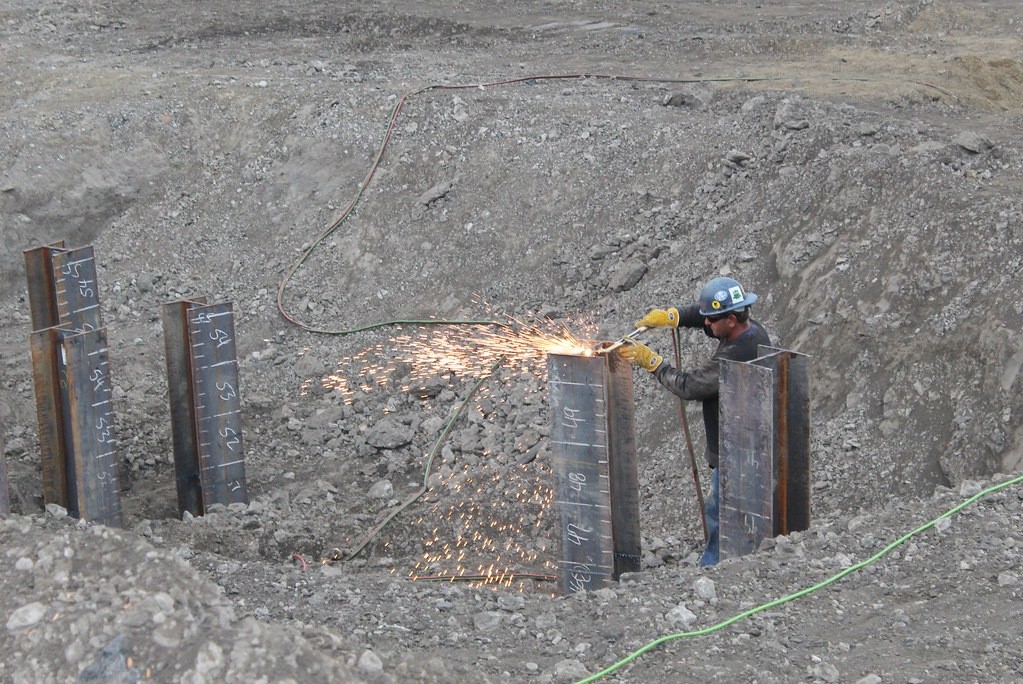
[706,311,734,323]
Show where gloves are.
[634,307,680,328]
[619,336,663,372]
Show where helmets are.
[699,277,758,315]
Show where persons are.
[617,277,771,565]
